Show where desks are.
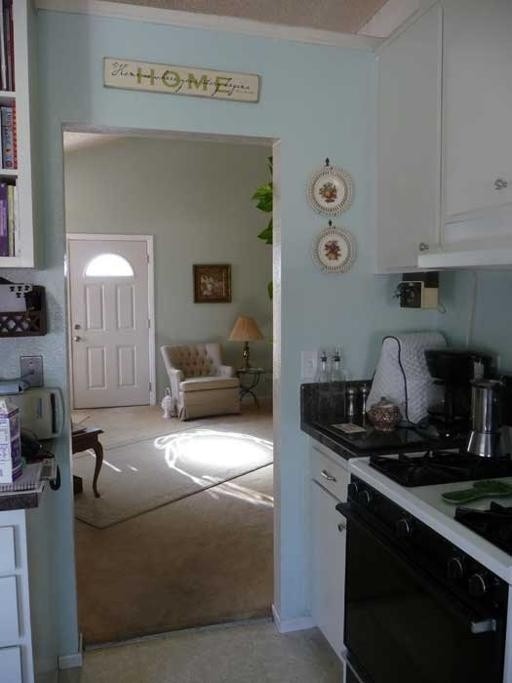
[72,423,104,498]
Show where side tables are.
[236,371,266,410]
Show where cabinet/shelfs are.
[0,458,58,683]
[442,1,511,253]
[300,430,351,683]
[372,0,444,271]
[0,0,45,269]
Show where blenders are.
[466,379,506,458]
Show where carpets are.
[72,412,274,530]
[35,620,344,683]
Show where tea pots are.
[370,396,402,434]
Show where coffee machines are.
[416,350,498,443]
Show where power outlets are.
[20,356,44,388]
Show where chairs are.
[160,344,241,421]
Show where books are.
[0,0,21,256]
[0,461,49,492]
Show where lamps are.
[228,316,264,371]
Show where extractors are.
[418,250,512,270]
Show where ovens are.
[335,473,512,683]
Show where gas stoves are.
[348,448,512,585]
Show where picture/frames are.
[192,263,232,304]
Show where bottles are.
[329,348,347,425]
[360,387,369,414]
[312,351,332,424]
[348,389,357,416]
